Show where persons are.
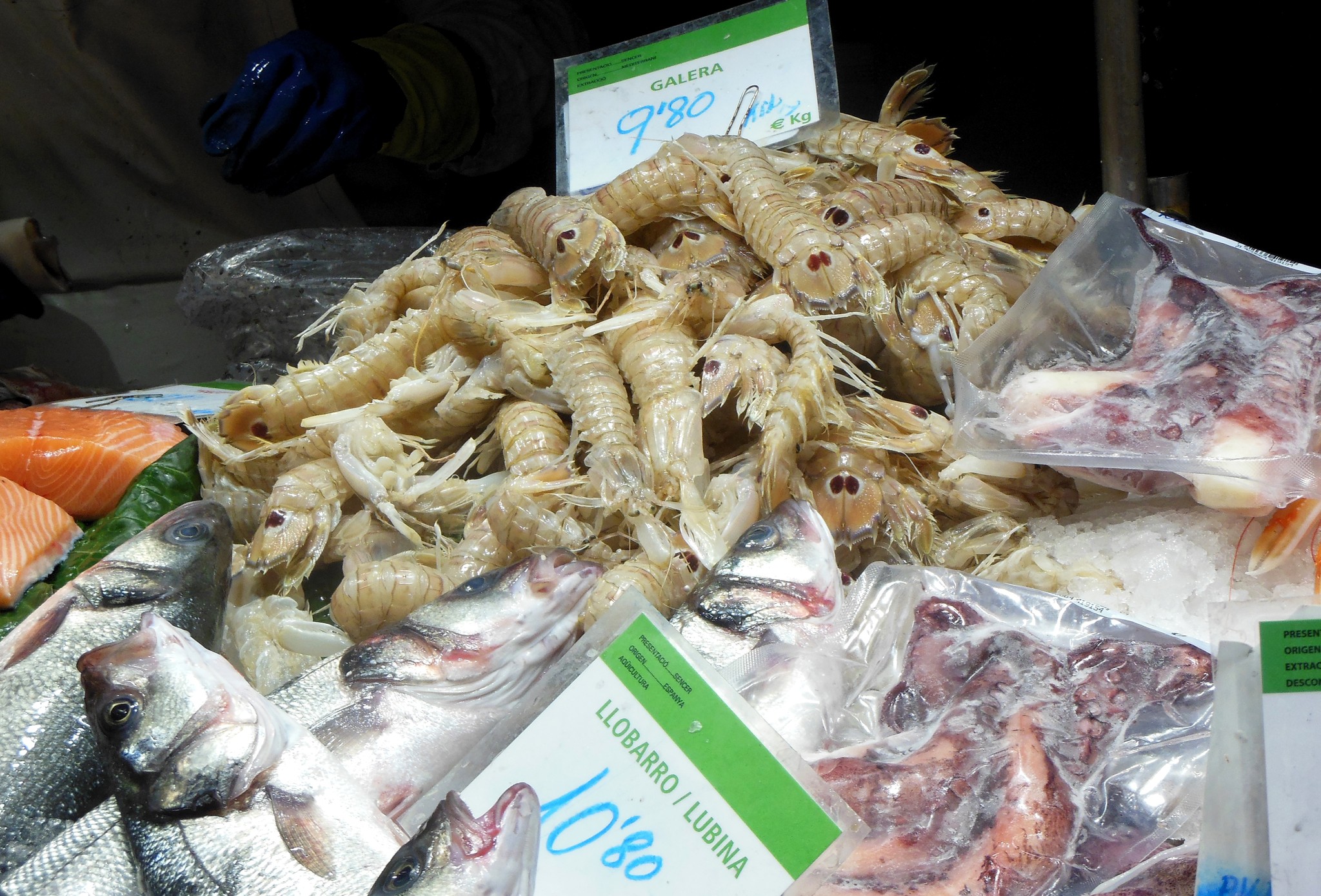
[4,4,597,306]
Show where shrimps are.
[178,61,1102,639]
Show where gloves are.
[194,26,408,199]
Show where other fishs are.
[0,496,844,896]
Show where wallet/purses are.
[0,215,72,294]
